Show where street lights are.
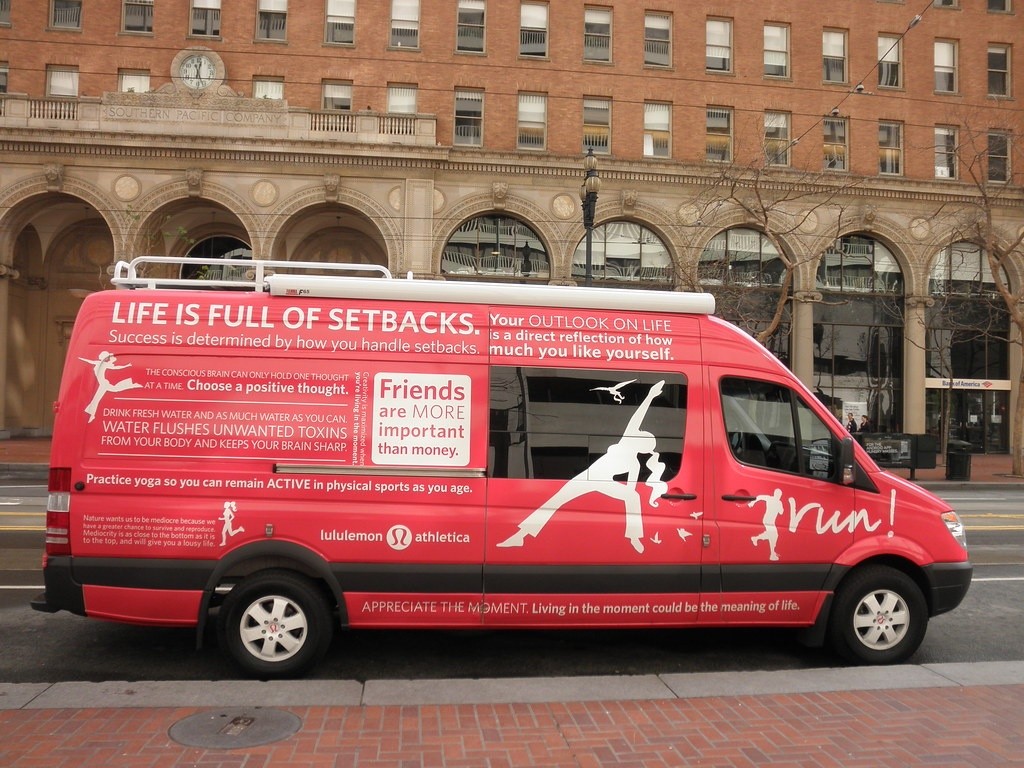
[575,146,606,289]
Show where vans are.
[30,256,968,663]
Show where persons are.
[845,413,857,433]
[858,415,871,433]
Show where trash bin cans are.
[946,437,974,481]
